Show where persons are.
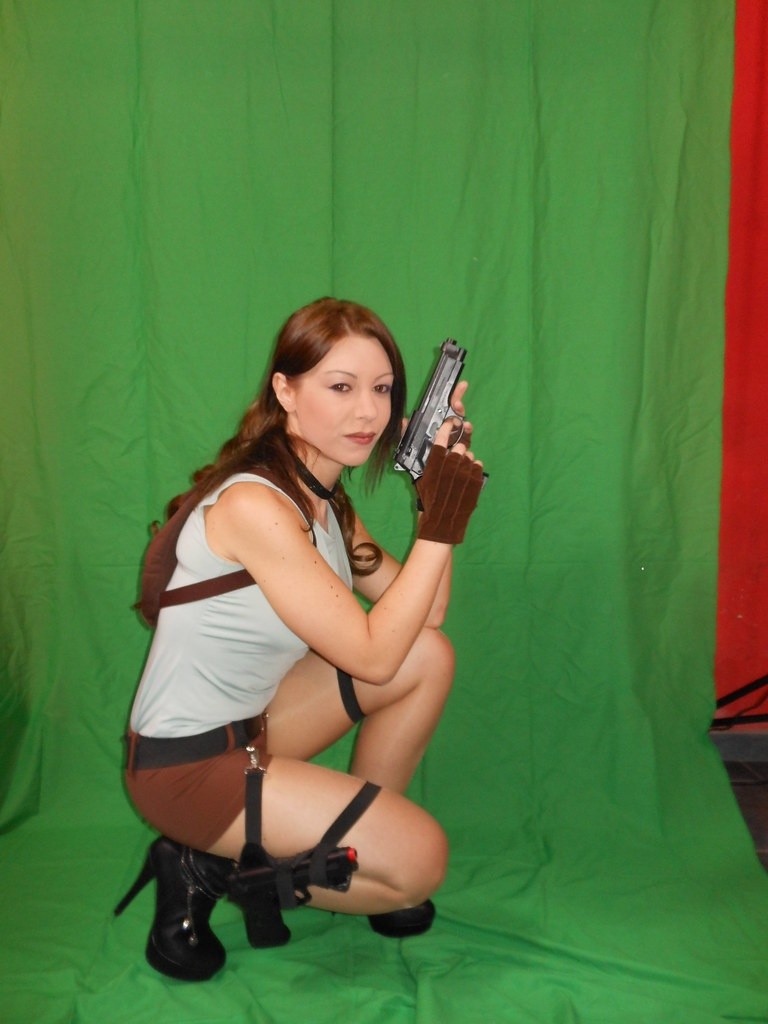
[112,297,483,984]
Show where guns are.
[221,845,360,952]
[390,334,490,496]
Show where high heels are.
[114,835,241,981]
[368,899,436,938]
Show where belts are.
[121,715,264,770]
